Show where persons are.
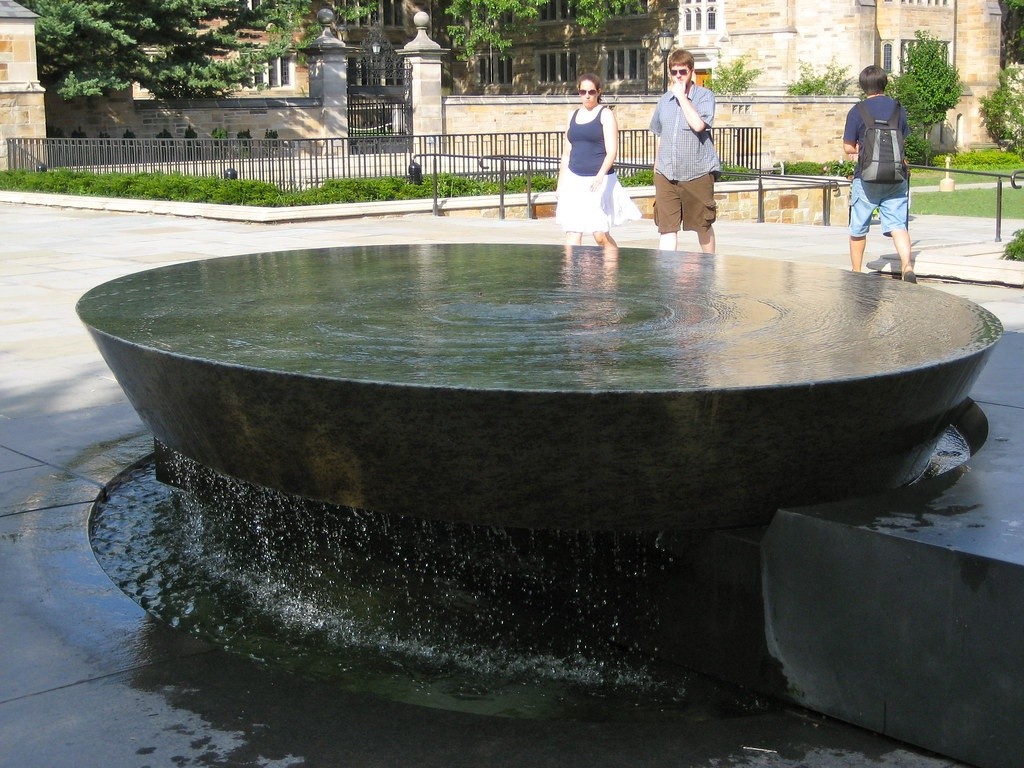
[843,66,917,282]
[557,72,642,248]
[650,50,721,252]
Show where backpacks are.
[856,100,907,184]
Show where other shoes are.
[903,271,916,283]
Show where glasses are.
[670,67,692,76]
[578,89,599,95]
